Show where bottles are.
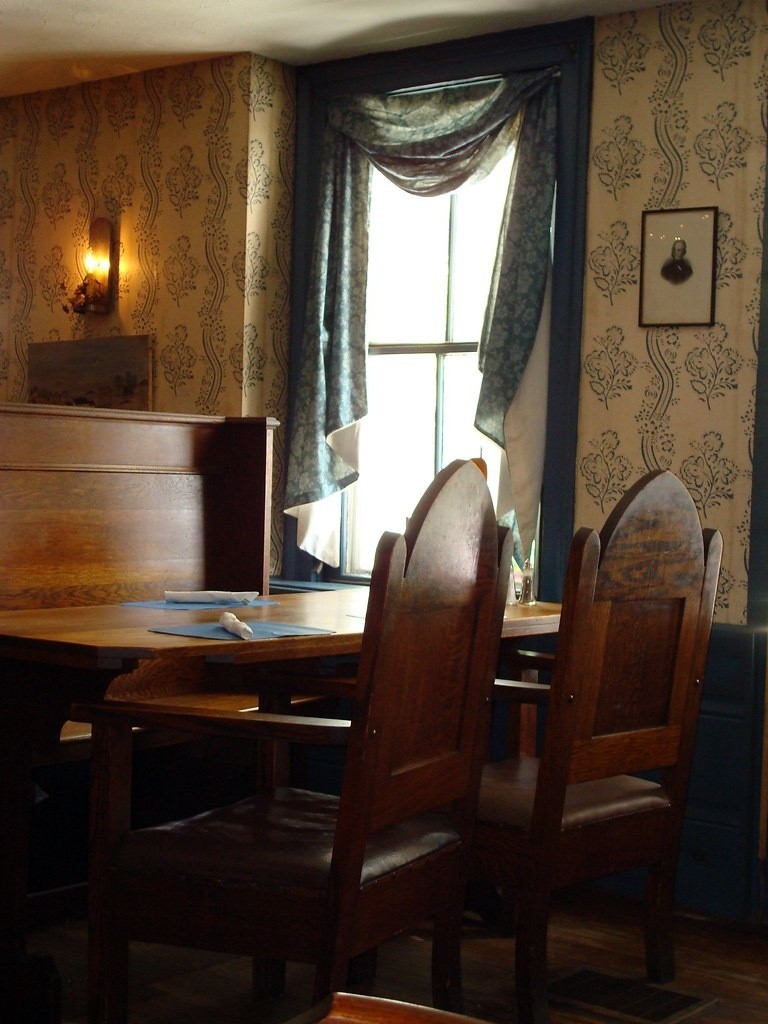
[517,567,537,607]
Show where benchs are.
[0,410,338,769]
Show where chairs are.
[64,456,507,1024]
[351,468,722,1024]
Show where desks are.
[0,592,565,1024]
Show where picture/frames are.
[638,205,719,327]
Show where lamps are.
[68,220,110,311]
